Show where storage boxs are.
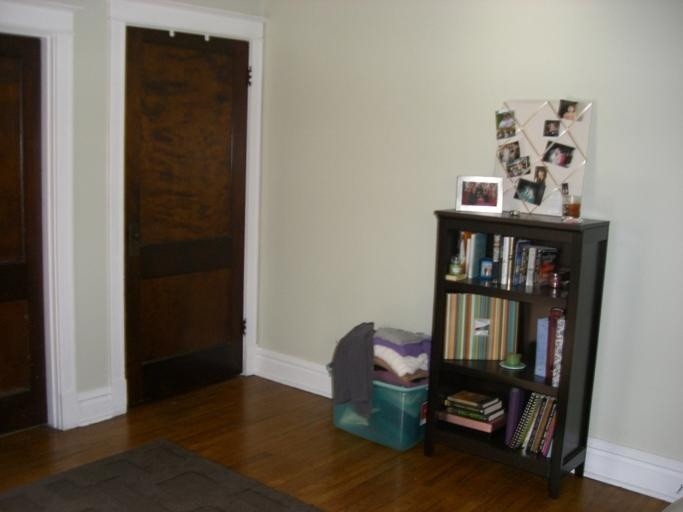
[326,359,429,453]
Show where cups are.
[562,194,582,219]
[505,352,522,367]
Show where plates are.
[499,361,527,370]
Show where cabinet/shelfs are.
[421,206,610,499]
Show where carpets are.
[1,439,332,512]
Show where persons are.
[494,100,584,206]
[462,182,497,206]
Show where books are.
[443,292,531,359]
[534,307,567,387]
[446,229,557,297]
[503,388,560,459]
[438,389,504,434]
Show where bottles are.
[549,272,561,289]
[450,255,463,276]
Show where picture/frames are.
[455,175,504,215]
